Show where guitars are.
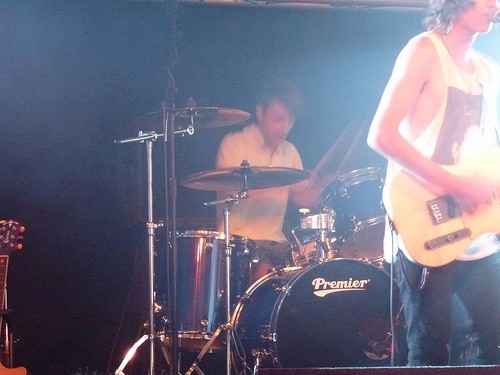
[0,219,28,374]
[390,152,499,268]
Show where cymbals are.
[133,105,252,133]
[179,164,312,193]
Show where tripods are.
[115,116,255,375]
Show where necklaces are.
[439,30,478,79]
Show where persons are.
[368,0,500,365]
[213,77,338,281]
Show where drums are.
[300,214,339,244]
[225,259,401,374]
[156,228,260,330]
[288,233,333,267]
[321,167,391,251]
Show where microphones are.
[488,7,500,23]
[357,336,386,351]
